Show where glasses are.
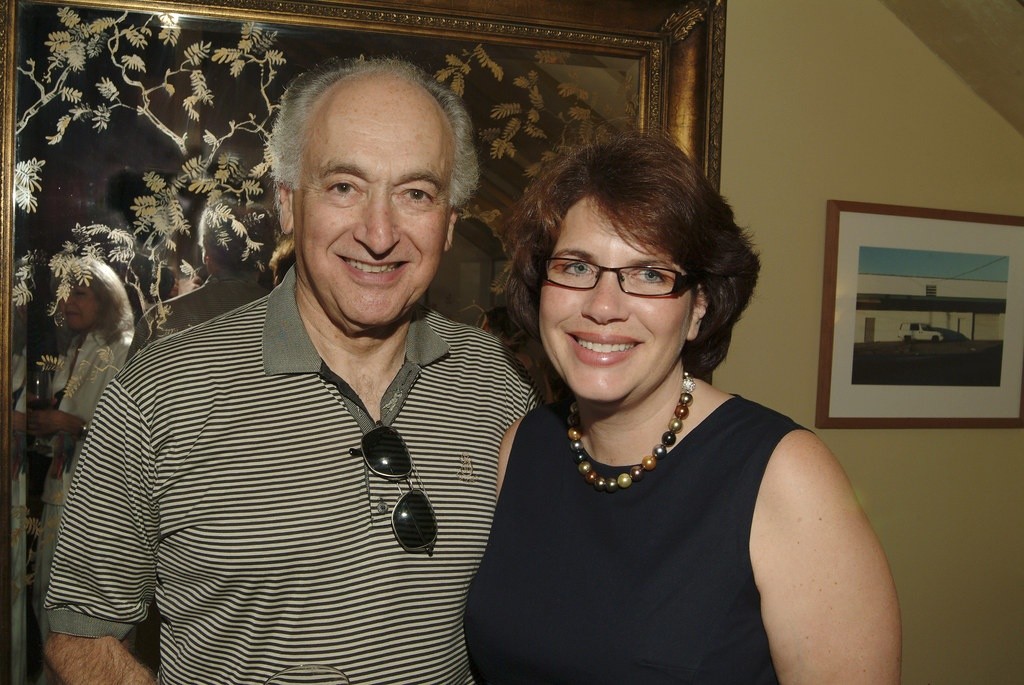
[539,257,695,297]
[348,420,438,556]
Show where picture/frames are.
[0,0,729,685]
[815,199,1024,429]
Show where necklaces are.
[567,372,696,493]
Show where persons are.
[463,131,901,685]
[479,303,564,404]
[24,199,275,640]
[42,56,547,685]
